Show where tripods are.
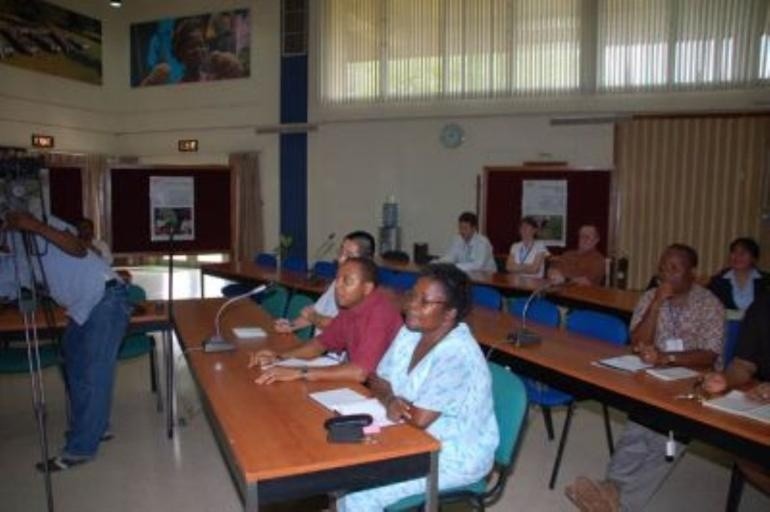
[0,208,75,512]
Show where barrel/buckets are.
[383,203,398,227]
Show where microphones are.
[201,284,271,353]
[304,232,335,283]
[506,282,560,346]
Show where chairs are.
[202,252,770,512]
[0,287,160,393]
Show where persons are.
[78,218,113,266]
[428,212,607,289]
[562,234,769,511]
[248,230,498,512]
[2,208,130,472]
[139,12,246,86]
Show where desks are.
[173,299,442,512]
[0,299,171,440]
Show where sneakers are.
[564,476,620,512]
[37,457,83,472]
